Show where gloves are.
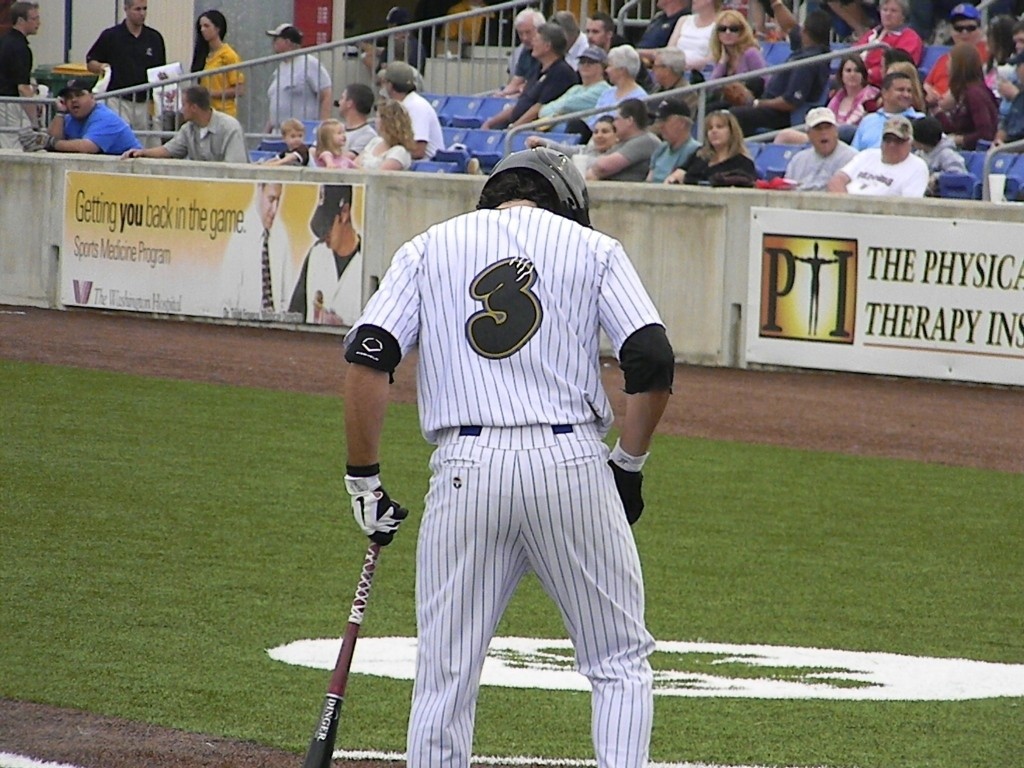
[18,128,56,152]
[346,463,408,546]
[608,460,644,525]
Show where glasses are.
[813,124,834,130]
[882,136,904,144]
[952,24,978,32]
[577,58,600,65]
[717,24,744,32]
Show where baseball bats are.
[302,498,400,768]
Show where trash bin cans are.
[30,63,58,92]
[46,65,101,97]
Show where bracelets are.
[345,463,380,477]
[753,99,758,108]
[54,111,65,117]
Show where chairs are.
[248,41,1024,203]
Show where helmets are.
[477,147,590,226]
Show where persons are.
[191,10,245,116]
[266,8,445,170]
[120,87,249,162]
[440,0,495,42]
[0,2,41,150]
[480,1,1024,199]
[345,149,674,768]
[220,183,361,326]
[266,23,331,129]
[18,79,144,154]
[86,0,166,145]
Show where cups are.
[987,174,1006,202]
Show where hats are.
[804,107,837,130]
[909,117,943,145]
[265,23,303,44]
[576,46,609,66]
[947,2,982,22]
[881,115,913,140]
[377,60,415,84]
[58,78,92,97]
[384,6,410,22]
[648,96,690,118]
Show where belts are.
[459,425,574,436]
